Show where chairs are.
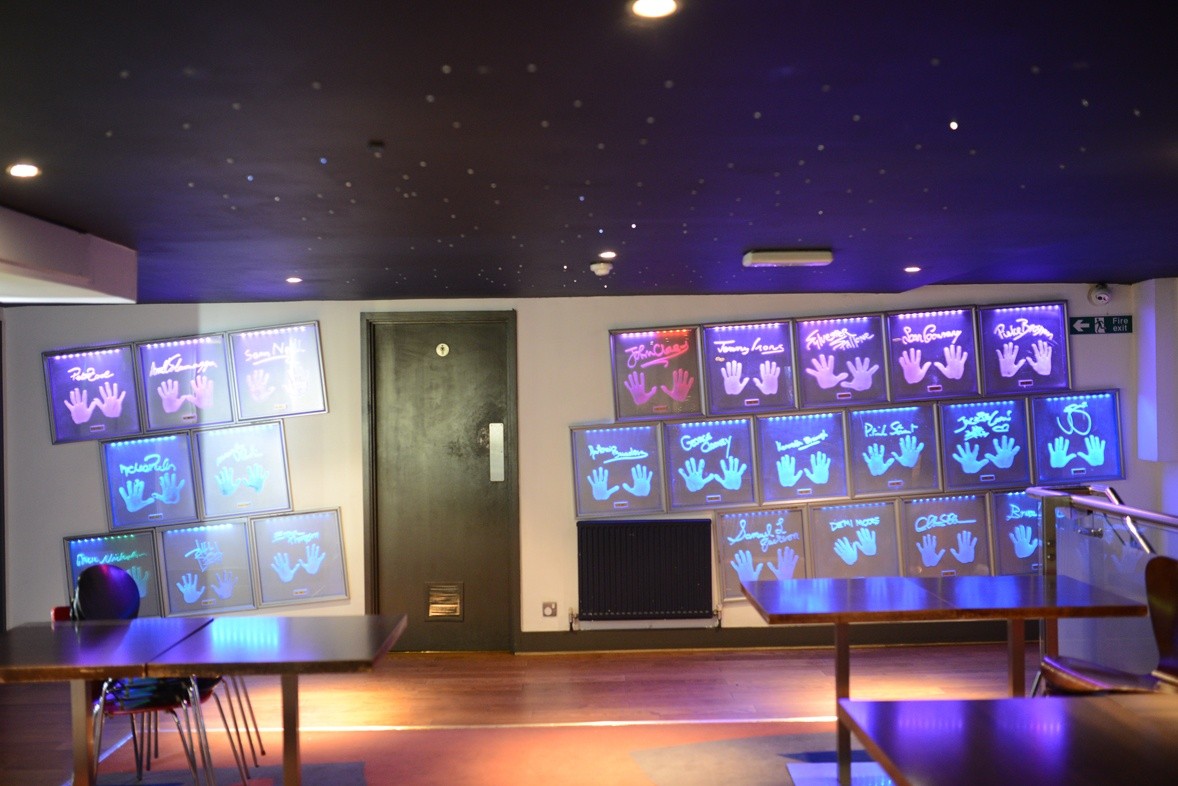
[70,564,265,786]
[1031,555,1178,697]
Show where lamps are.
[744,251,832,266]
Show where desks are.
[1,613,408,779]
[739,575,1178,785]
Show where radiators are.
[568,511,721,631]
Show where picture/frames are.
[41,320,350,622]
[569,300,1127,605]
